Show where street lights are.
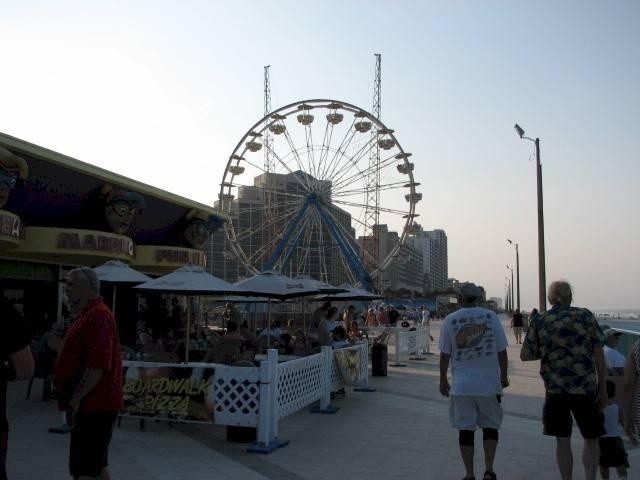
[504,239,520,315]
[515,123,547,312]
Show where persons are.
[599,379,631,480]
[41,319,63,400]
[527,308,538,327]
[436,286,509,480]
[519,282,610,480]
[602,328,625,377]
[53,266,125,480]
[1,293,36,479]
[622,337,640,445]
[511,308,524,344]
[161,295,431,371]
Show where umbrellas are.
[132,260,250,364]
[218,295,298,339]
[235,267,320,351]
[94,259,155,316]
[312,282,384,301]
[293,273,352,333]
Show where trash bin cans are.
[227,361,258,443]
[522,314,528,331]
[372,344,388,376]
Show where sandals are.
[482,470,497,480]
[462,475,475,480]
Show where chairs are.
[25,327,390,430]
